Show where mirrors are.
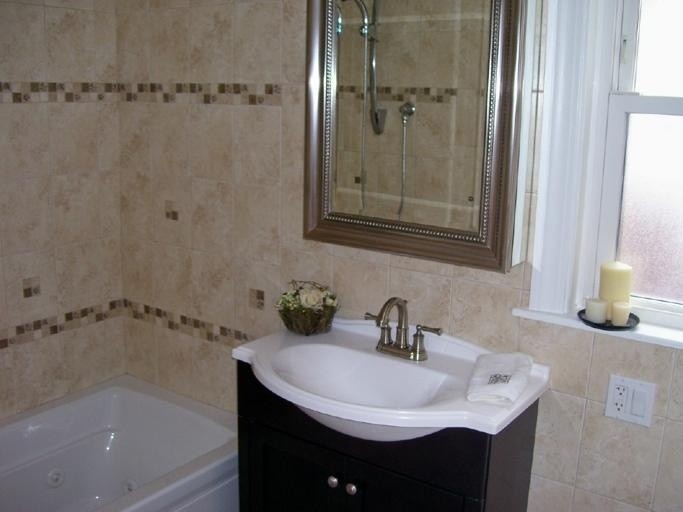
[303,0,528,273]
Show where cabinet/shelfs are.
[237,360,538,512]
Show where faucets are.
[365,294,443,362]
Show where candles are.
[585,260,633,327]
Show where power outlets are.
[605,375,657,427]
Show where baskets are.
[278,277,336,336]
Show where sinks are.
[230,317,551,445]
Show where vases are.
[278,308,336,336]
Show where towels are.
[466,352,531,406]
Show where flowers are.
[274,279,338,316]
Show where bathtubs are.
[1,371,240,512]
